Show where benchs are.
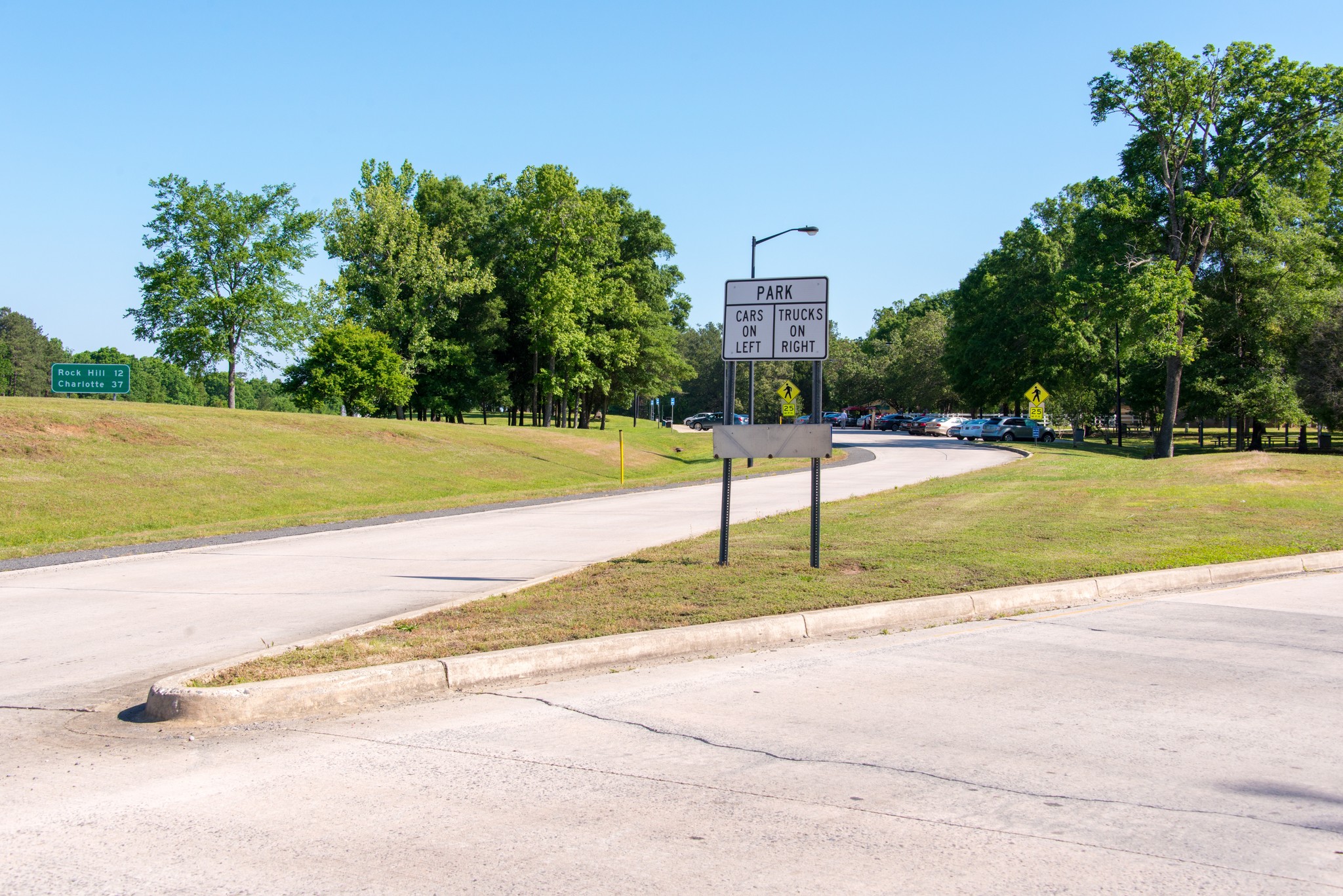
[1121,430,1156,437]
[1211,440,1252,448]
[1262,440,1300,448]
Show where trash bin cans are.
[1076,428,1085,442]
[1121,423,1127,435]
[655,415,660,422]
[1320,434,1331,448]
[666,417,672,428]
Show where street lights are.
[747,224,819,469]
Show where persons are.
[864,416,872,430]
[840,410,847,429]
[898,408,903,414]
[921,412,926,416]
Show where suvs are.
[980,417,1056,442]
[808,411,842,423]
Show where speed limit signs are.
[782,404,795,416]
[1030,407,1043,420]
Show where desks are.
[1126,426,1153,437]
[1262,435,1302,447]
[1212,435,1250,446]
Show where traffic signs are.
[1030,426,1042,428]
[656,399,659,405]
[651,400,653,405]
[722,275,830,361]
[671,398,675,405]
[1033,428,1039,437]
[52,362,131,395]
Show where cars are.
[910,417,949,436]
[857,414,883,429]
[925,417,971,437]
[739,415,749,421]
[960,419,990,441]
[949,419,980,440]
[794,416,810,424]
[874,414,915,431]
[683,413,723,426]
[824,413,858,427]
[689,412,749,431]
[899,416,933,435]
